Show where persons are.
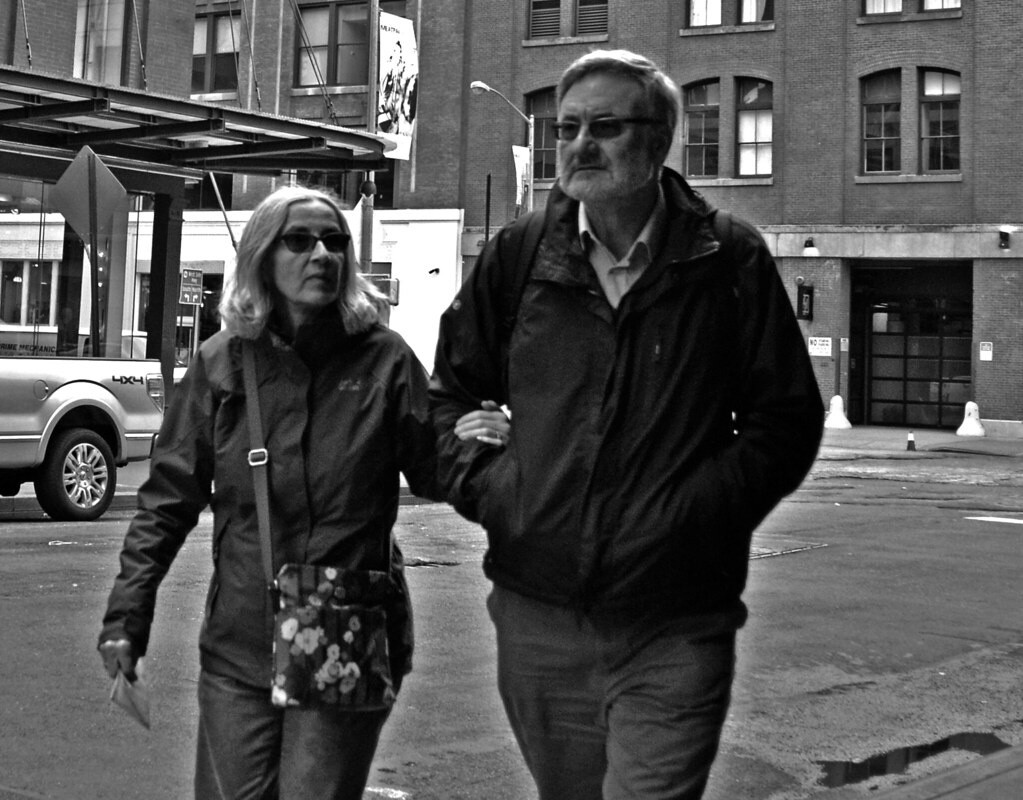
[383,42,406,135]
[96,186,510,800]
[428,51,825,800]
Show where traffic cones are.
[905,429,917,449]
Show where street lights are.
[469,80,534,214]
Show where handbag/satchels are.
[269,563,396,713]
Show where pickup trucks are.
[0,353,166,519]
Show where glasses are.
[278,233,352,253]
[550,116,665,141]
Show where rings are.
[496,431,503,439]
